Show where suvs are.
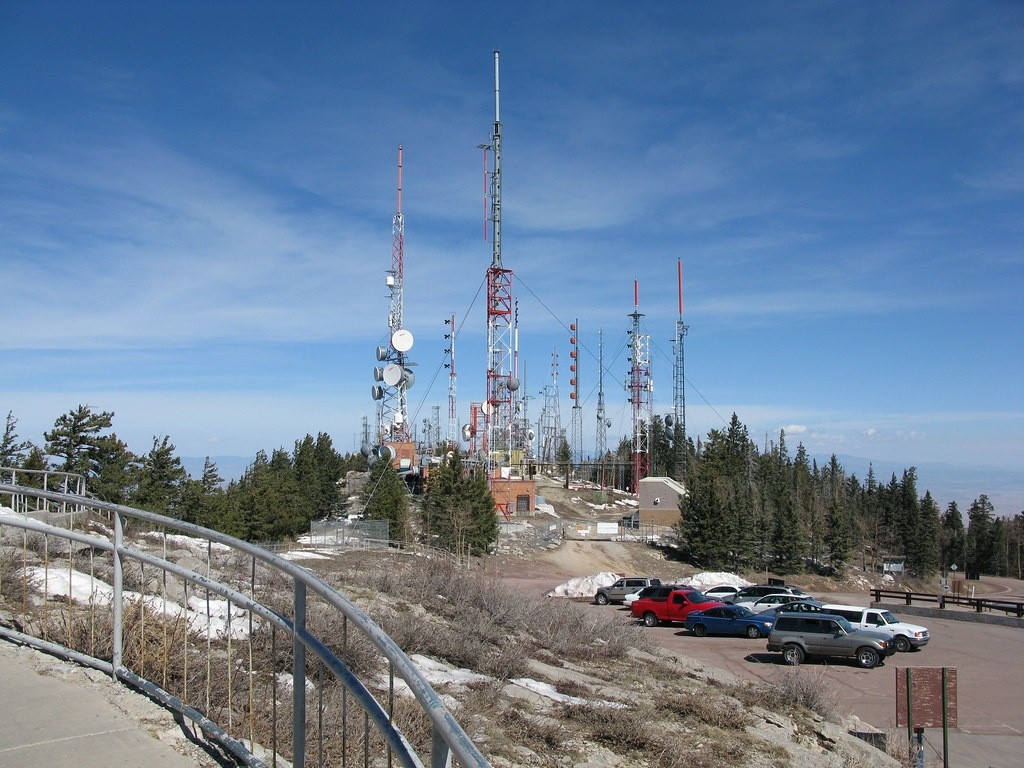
[720,584,801,606]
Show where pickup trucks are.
[594,577,651,606]
[821,604,930,653]
[629,590,726,627]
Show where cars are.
[701,584,746,599]
[684,605,775,640]
[639,579,707,602]
[622,586,645,606]
[766,611,897,668]
[758,600,829,617]
